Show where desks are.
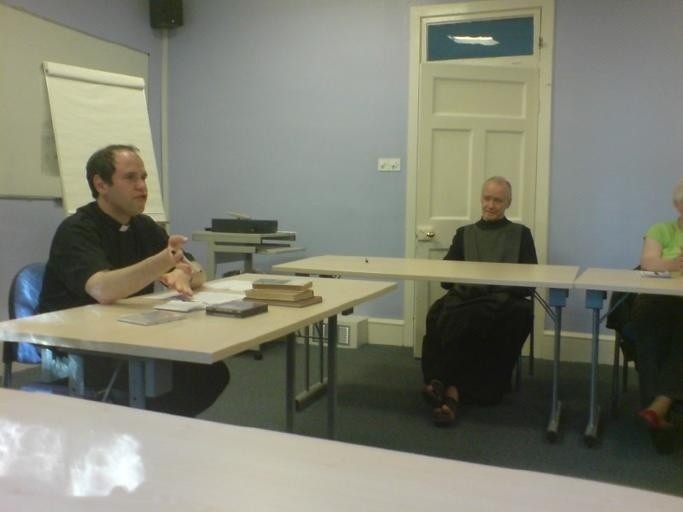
[573,262,680,450]
[0,385,680,511]
[2,272,402,443]
[269,250,580,438]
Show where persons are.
[34,144,230,416]
[637,178,683,431]
[421,176,538,427]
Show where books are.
[205,277,322,319]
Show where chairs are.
[2,262,97,398]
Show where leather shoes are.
[637,403,673,435]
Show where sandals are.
[422,378,460,424]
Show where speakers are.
[149,0,183,29]
